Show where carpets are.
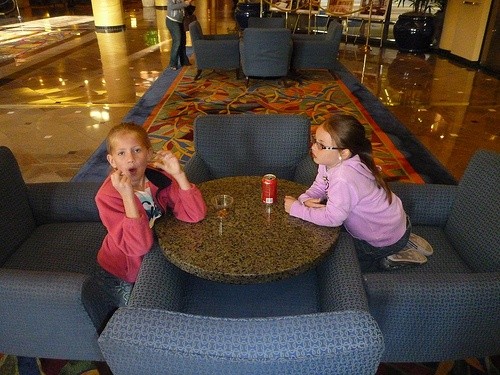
[1,47,500,375]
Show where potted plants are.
[392,0,447,53]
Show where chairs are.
[0,145,118,359]
[248,17,286,29]
[189,20,241,81]
[238,29,293,88]
[357,150,500,375]
[183,114,319,187]
[97,232,386,375]
[289,21,344,82]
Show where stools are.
[270,10,385,48]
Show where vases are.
[234,2,267,31]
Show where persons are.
[95,122,207,306]
[284,115,435,270]
[167,0,194,71]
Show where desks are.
[155,175,340,285]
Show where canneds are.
[261,174,278,204]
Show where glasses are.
[310,135,347,151]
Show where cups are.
[211,195,233,222]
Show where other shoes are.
[168,66,178,71]
[405,232,434,256]
[181,63,192,67]
[384,248,428,268]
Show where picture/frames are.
[326,0,354,16]
[270,0,293,13]
[359,0,389,20]
[297,0,321,13]
